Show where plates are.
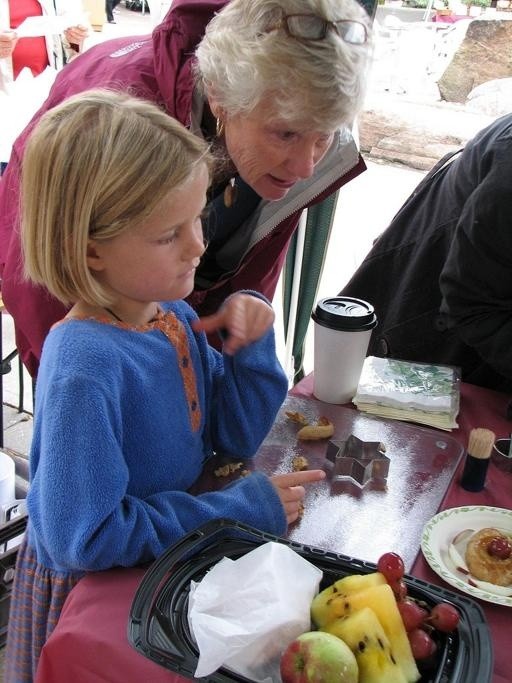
[420,504,512,607]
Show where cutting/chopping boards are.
[187,395,466,575]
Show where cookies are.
[300,417,333,441]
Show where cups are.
[0,452,16,510]
[310,296,378,404]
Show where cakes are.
[466,529,511,585]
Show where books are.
[354,355,460,431]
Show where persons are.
[0,0,94,82]
[4,85,326,683]
[342,110,512,404]
[2,0,374,380]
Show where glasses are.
[257,13,368,46]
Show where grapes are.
[376,550,455,659]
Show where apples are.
[281,631,360,683]
